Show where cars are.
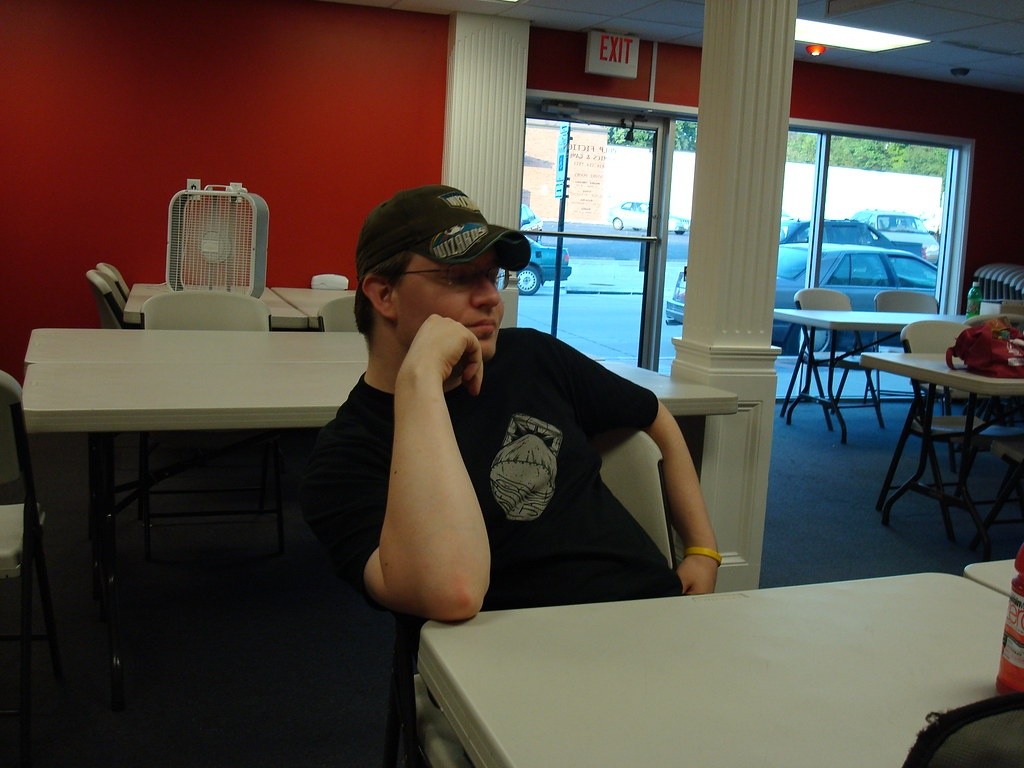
[606,200,691,235]
[665,241,940,354]
[516,235,572,296]
[851,209,940,266]
[778,218,927,260]
[521,203,543,244]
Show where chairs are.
[381,611,472,768]
[863,290,939,424]
[779,288,885,428]
[874,315,1024,550]
[0,263,367,767]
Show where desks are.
[772,308,965,445]
[19,282,742,710]
[860,353,1024,562]
[963,559,1019,598]
[418,573,1009,768]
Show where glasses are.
[381,261,510,291]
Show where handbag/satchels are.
[946,316,1024,378]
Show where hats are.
[354,185,531,291]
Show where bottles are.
[996,542,1024,696]
[965,282,982,321]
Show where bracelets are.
[684,546,722,567]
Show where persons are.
[296,186,721,709]
